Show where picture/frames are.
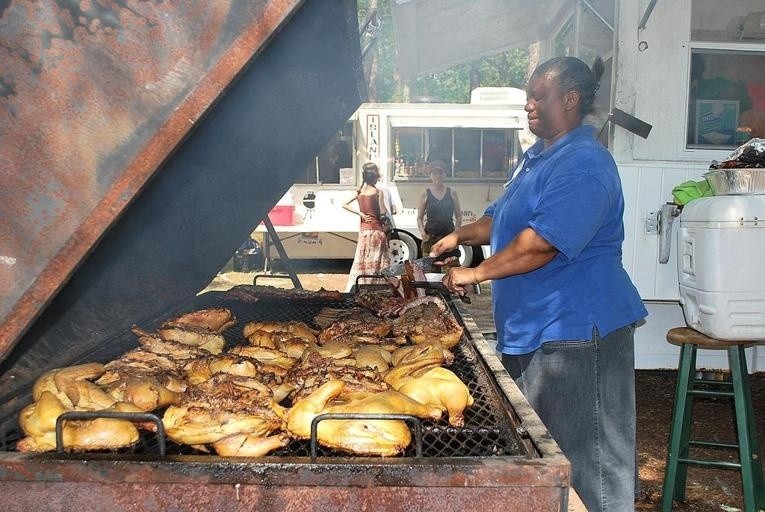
[695,98,739,148]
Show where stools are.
[659,327,764,511]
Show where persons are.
[430,57,649,512]
[342,168,390,294]
[361,163,400,240]
[687,53,754,144]
[417,160,470,296]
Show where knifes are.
[380,249,461,277]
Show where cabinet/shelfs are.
[683,38,765,152]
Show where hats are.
[428,160,446,173]
